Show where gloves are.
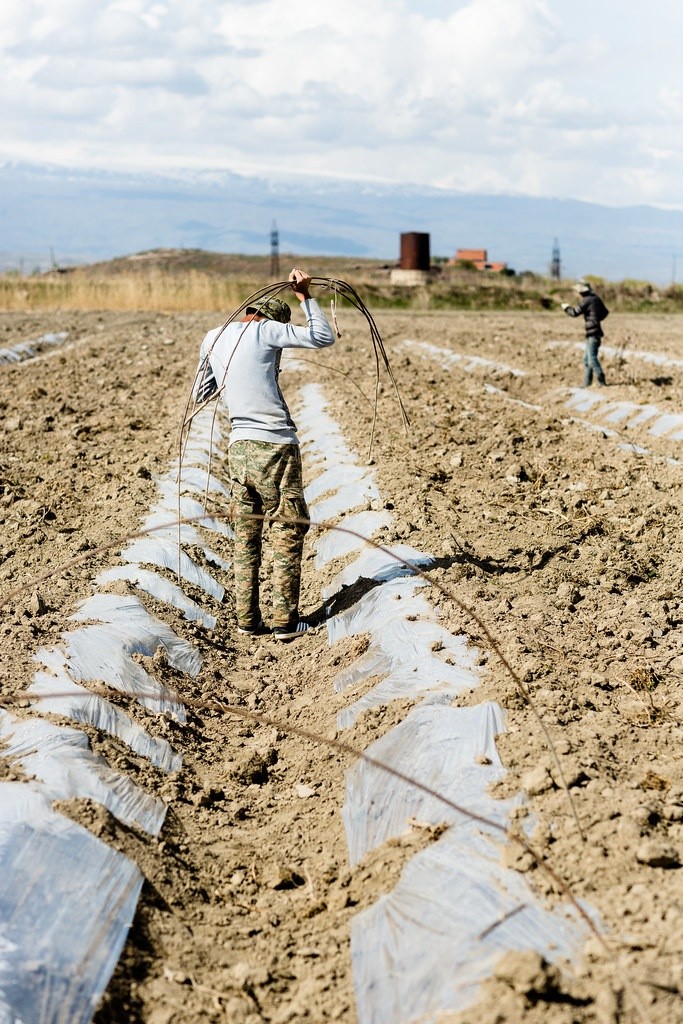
[560,303,569,310]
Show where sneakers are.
[237,620,262,634]
[273,619,320,639]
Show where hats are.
[572,280,591,293]
[245,296,290,323]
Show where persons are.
[560,279,608,388]
[192,268,336,639]
